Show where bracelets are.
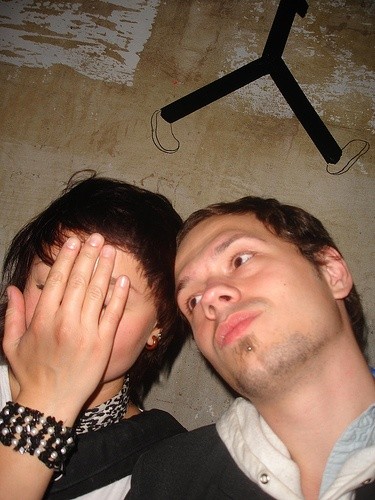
[0,400,82,471]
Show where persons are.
[0,179,194,500]
[125,198,375,500]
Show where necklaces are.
[72,373,132,437]
